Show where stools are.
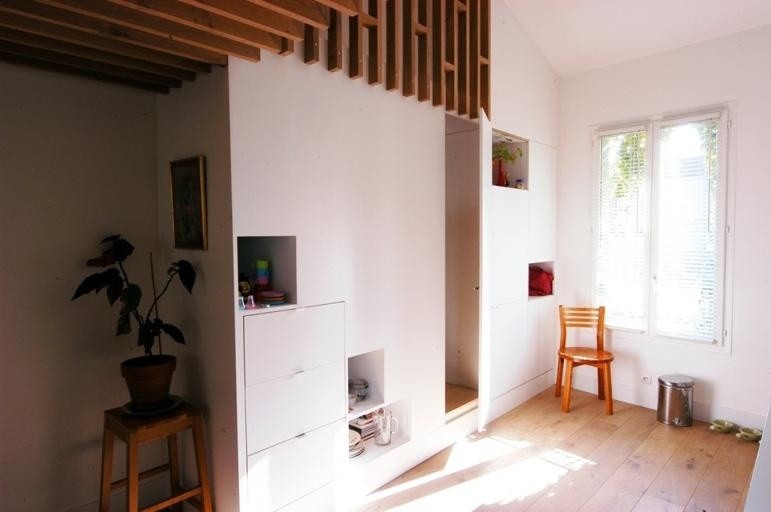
[99,403,213,512]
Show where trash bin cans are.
[657,375,695,426]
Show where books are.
[349,410,383,441]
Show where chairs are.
[555,306,614,415]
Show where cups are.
[372,409,399,447]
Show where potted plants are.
[492,138,523,186]
[71,234,197,415]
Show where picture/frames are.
[170,156,206,250]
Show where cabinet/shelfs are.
[444,108,489,433]
[244,302,348,512]
